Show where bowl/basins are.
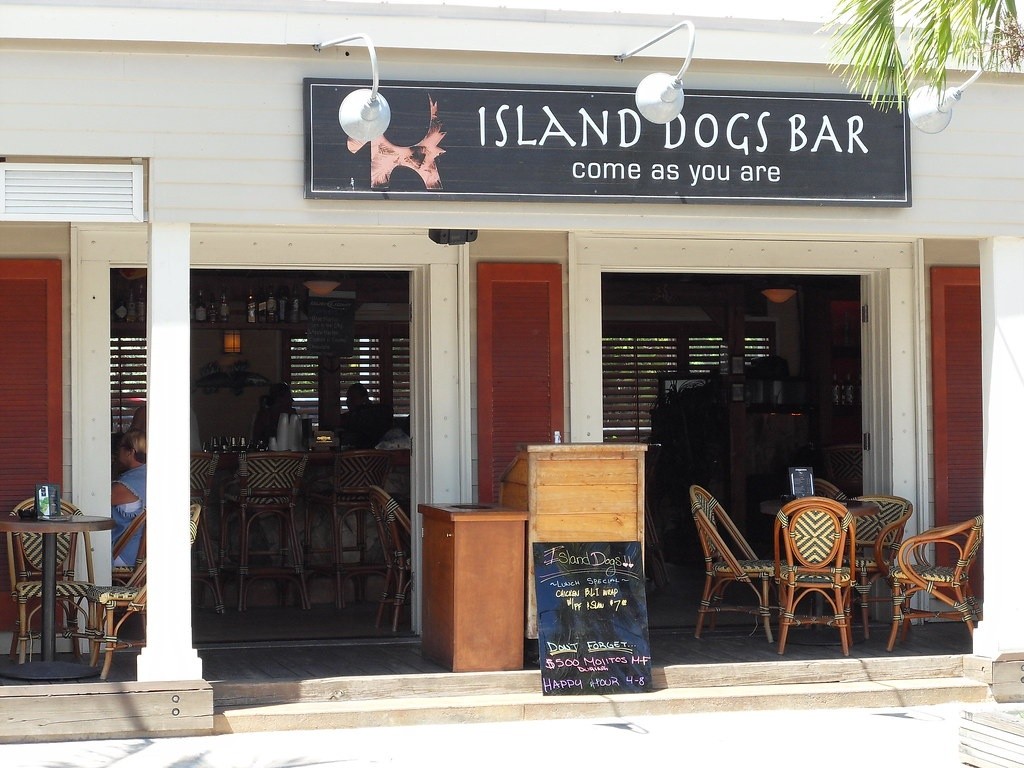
[18,509,35,520]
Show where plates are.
[42,518,69,522]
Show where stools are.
[643,442,670,585]
[188,452,224,612]
[220,450,311,612]
[299,448,394,609]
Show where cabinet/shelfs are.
[417,503,528,672]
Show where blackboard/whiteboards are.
[531,540,655,697]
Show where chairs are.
[87,505,203,679]
[774,498,857,655]
[365,483,412,634]
[813,444,865,501]
[809,495,914,640]
[5,498,97,666]
[686,484,785,644]
[884,514,983,653]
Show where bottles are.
[267,293,278,322]
[831,371,840,407]
[841,373,854,408]
[247,288,256,323]
[190,292,195,322]
[195,290,206,322]
[127,288,137,322]
[207,294,217,322]
[136,284,146,321]
[219,292,230,322]
[289,296,300,322]
[114,294,127,322]
[279,295,287,320]
[259,295,266,322]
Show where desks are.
[0,515,116,680]
[762,502,879,645]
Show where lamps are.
[316,34,391,140]
[614,18,696,125]
[907,36,994,134]
[302,278,341,295]
[223,330,241,353]
[761,278,798,304]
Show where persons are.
[111,407,146,567]
[253,386,299,442]
[313,383,395,495]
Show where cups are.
[269,437,277,450]
[289,414,302,451]
[276,413,290,451]
